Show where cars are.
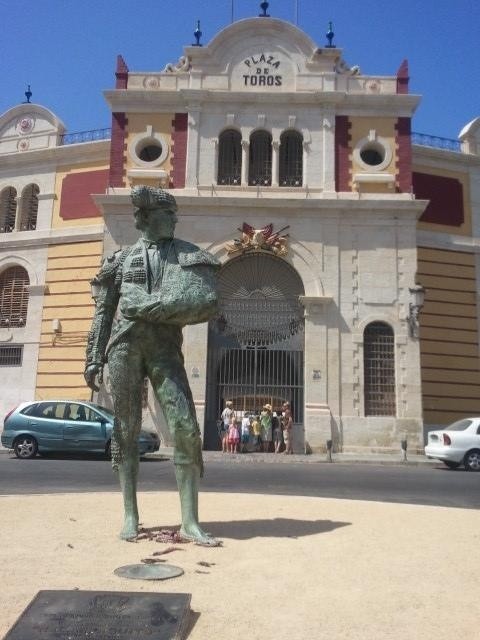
[1,400,161,464]
[421,418,480,470]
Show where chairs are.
[76,405,86,421]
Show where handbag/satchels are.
[217,420,223,430]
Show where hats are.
[263,404,272,411]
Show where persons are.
[220,400,293,455]
[83,183,222,547]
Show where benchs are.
[47,410,55,418]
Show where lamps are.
[407,281,425,338]
